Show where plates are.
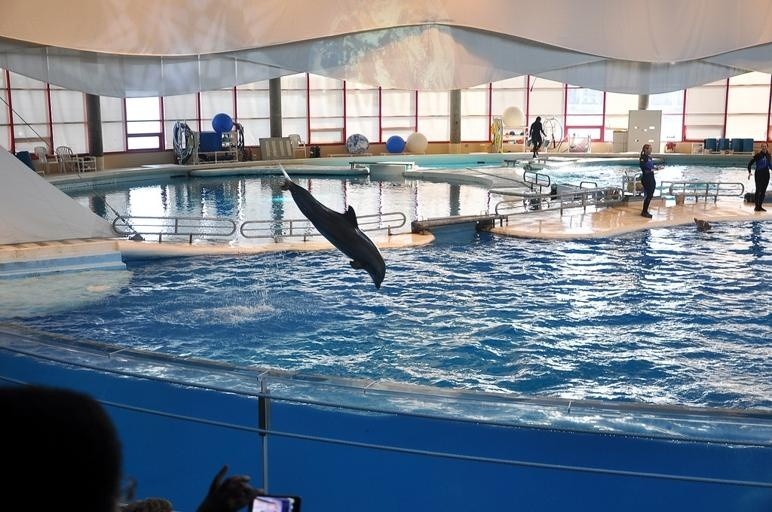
[501,126,530,152]
[193,131,239,164]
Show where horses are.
[247,494,302,512]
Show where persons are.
[2,380,126,509]
[638,144,656,218]
[746,142,771,212]
[528,116,547,158]
[195,463,265,510]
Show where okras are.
[278,162,386,289]
[694,217,712,232]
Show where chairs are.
[755,207,766,211]
[533,149,538,158]
[641,211,652,218]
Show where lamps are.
[15,145,81,178]
[289,134,307,158]
